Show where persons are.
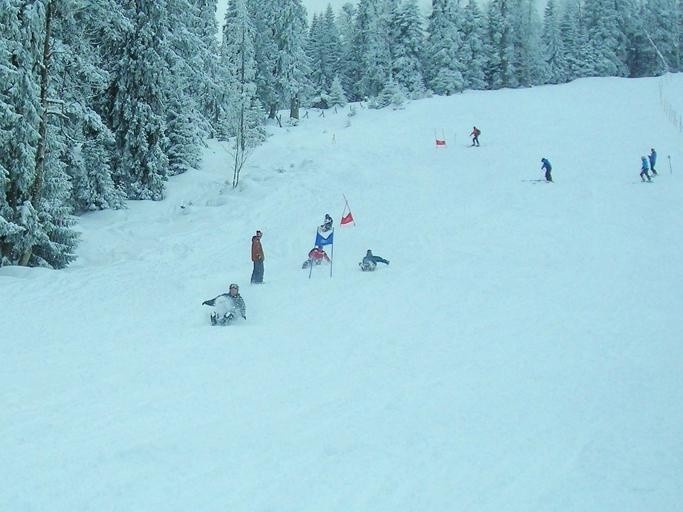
[302,245,331,269]
[360,249,389,271]
[639,156,651,182]
[202,284,246,325]
[321,214,333,232]
[251,232,264,284]
[469,126,479,146]
[648,148,657,176]
[541,157,552,181]
